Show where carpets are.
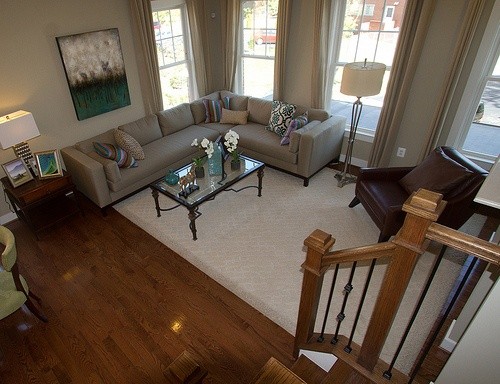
[112,167,488,376]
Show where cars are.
[255,29,276,45]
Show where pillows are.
[280,111,309,147]
[203,96,231,123]
[114,129,145,160]
[220,108,249,125]
[264,100,298,137]
[93,142,138,168]
[399,147,475,196]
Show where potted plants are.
[229,148,243,170]
[191,158,208,179]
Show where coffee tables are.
[149,153,265,241]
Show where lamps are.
[0,110,40,170]
[334,59,386,187]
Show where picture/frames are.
[34,149,64,180]
[2,158,35,189]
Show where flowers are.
[191,136,215,159]
[223,130,240,154]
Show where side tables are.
[0,168,83,241]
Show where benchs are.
[222,98,347,187]
[60,103,220,216]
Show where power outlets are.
[398,147,406,158]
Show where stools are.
[190,91,252,135]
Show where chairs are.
[348,147,490,243]
[0,225,46,323]
